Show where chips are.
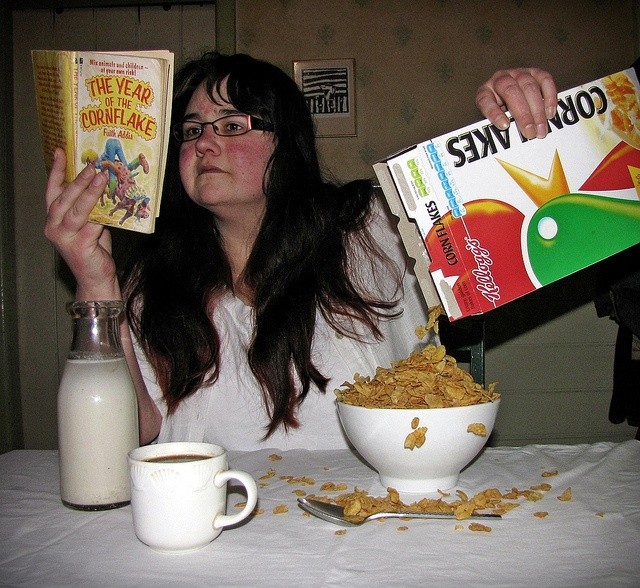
[335,303,500,450]
[234,455,571,535]
[596,72,640,151]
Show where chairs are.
[440,316,485,389]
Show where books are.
[21,45,176,235]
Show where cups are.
[127,441,257,548]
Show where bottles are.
[57,300,137,513]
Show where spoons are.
[297,498,503,528]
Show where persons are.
[45,50,558,450]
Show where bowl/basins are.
[337,401,500,494]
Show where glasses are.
[171,113,276,143]
